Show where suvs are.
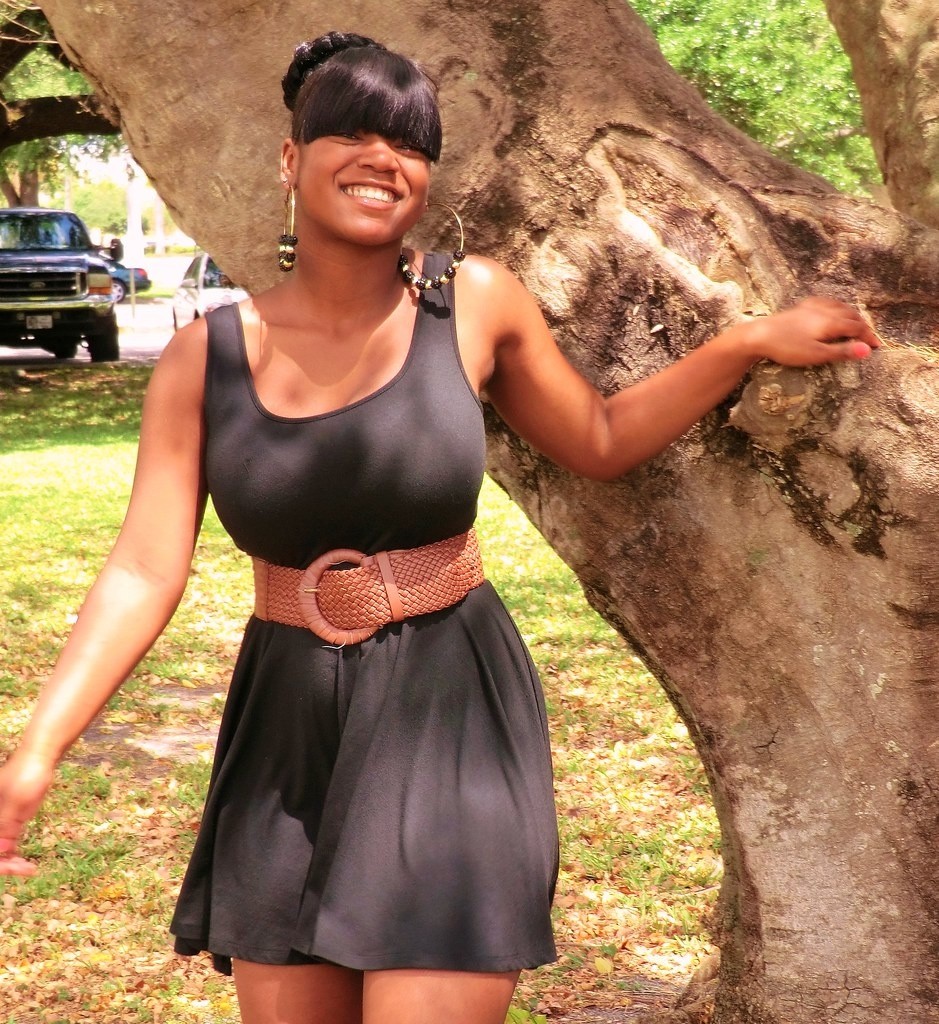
[1,207,125,363]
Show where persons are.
[0,29,880,1024]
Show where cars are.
[171,253,251,334]
[100,254,151,304]
